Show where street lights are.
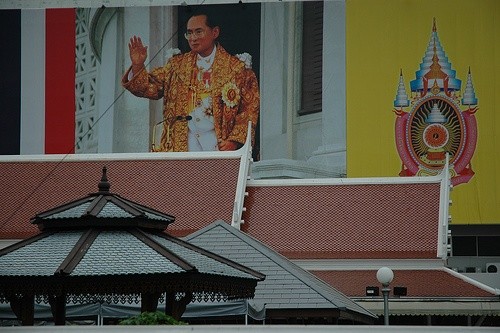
[376,267,395,324]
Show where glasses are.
[182,31,208,40]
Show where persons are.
[118,6,259,158]
